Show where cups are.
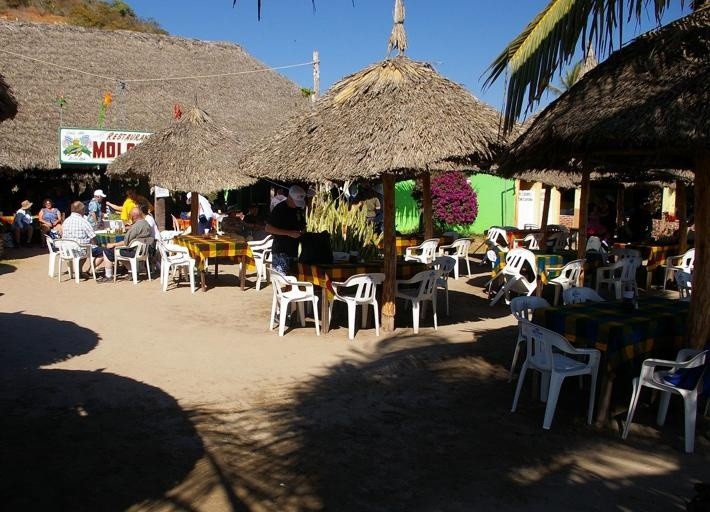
[120,227,123,234]
[204,229,209,238]
[107,227,110,234]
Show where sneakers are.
[81,272,114,283]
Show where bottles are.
[621,279,635,306]
[215,234,218,241]
[115,221,120,234]
[349,250,358,264]
[106,205,109,214]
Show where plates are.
[335,263,353,267]
[370,259,384,263]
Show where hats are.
[94,190,107,198]
[290,185,306,207]
[186,192,192,204]
[21,200,33,210]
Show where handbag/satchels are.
[298,230,334,265]
[199,214,207,223]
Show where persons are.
[265,184,334,327]
[96,200,160,283]
[13,200,33,245]
[87,189,107,229]
[186,192,213,234]
[361,197,381,226]
[220,203,273,241]
[120,186,155,225]
[60,200,105,277]
[37,199,61,245]
